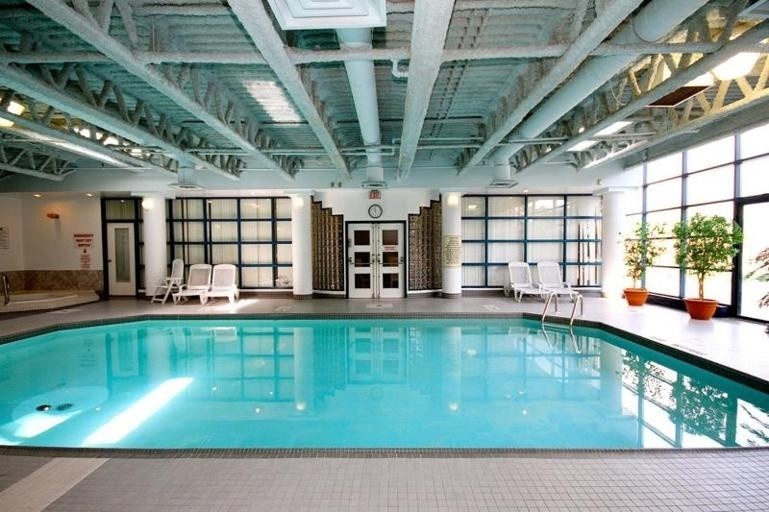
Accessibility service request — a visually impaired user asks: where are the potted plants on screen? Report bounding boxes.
[622,224,666,307]
[672,211,743,320]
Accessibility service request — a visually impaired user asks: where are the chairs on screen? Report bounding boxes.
[537,260,580,303]
[172,262,212,307]
[203,263,240,305]
[507,261,549,303]
[149,259,186,307]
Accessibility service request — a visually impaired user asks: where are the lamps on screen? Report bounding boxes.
[710,22,768,81]
[0,96,27,128]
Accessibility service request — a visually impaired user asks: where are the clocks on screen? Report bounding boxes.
[368,204,382,218]
